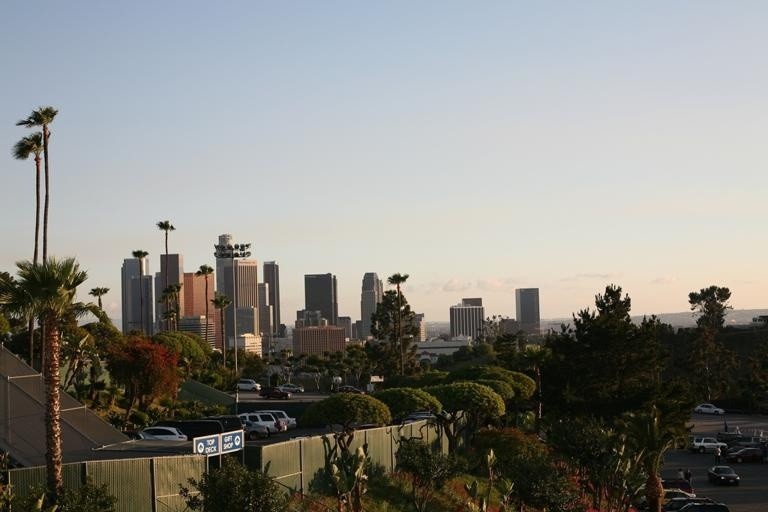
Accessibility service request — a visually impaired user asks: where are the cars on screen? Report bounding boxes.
[259,383,304,400]
[335,385,361,394]
[708,465,740,486]
[688,426,768,463]
[134,409,297,442]
[661,478,730,512]
[694,403,726,415]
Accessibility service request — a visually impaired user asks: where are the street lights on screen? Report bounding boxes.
[213,244,251,377]
[333,375,342,394]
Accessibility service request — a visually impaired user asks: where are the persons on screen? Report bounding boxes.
[678,469,683,480]
[685,468,692,484]
[713,445,721,465]
[721,446,728,464]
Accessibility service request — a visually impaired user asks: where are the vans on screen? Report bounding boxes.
[236,378,261,392]
